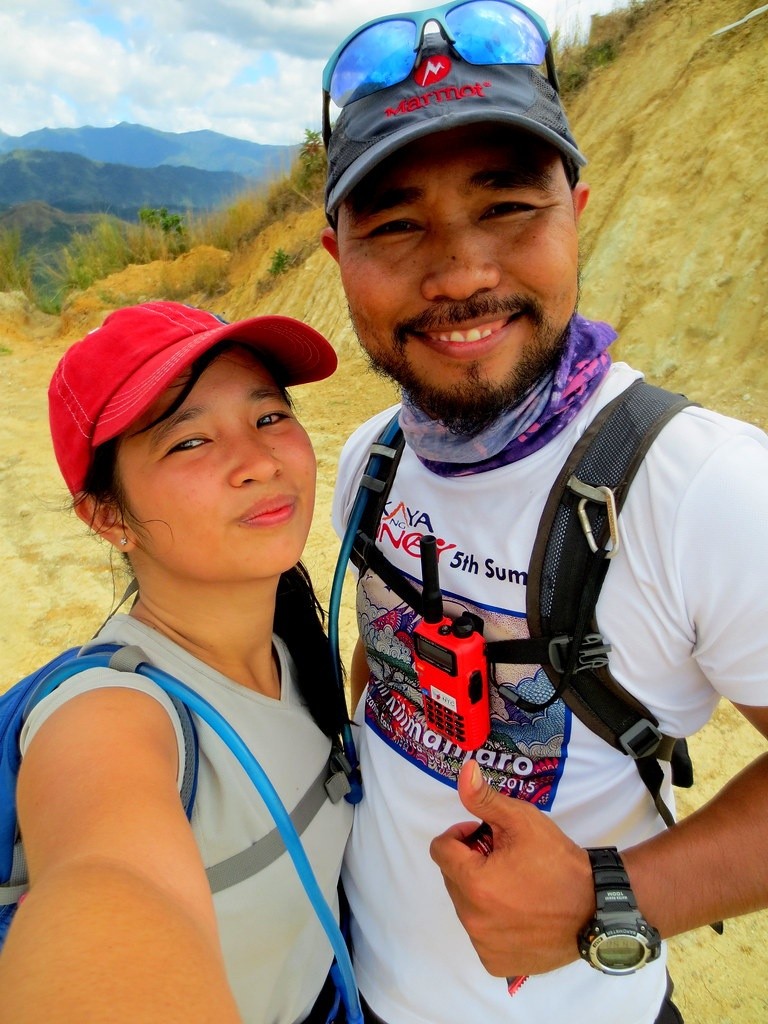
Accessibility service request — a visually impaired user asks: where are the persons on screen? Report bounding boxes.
[320,0,768,1024]
[0,299,363,1024]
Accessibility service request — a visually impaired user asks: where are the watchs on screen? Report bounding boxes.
[575,846,660,976]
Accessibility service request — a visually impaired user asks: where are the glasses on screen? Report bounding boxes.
[322,0,559,158]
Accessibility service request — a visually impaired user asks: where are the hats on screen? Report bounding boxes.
[325,32,588,233]
[49,301,338,494]
